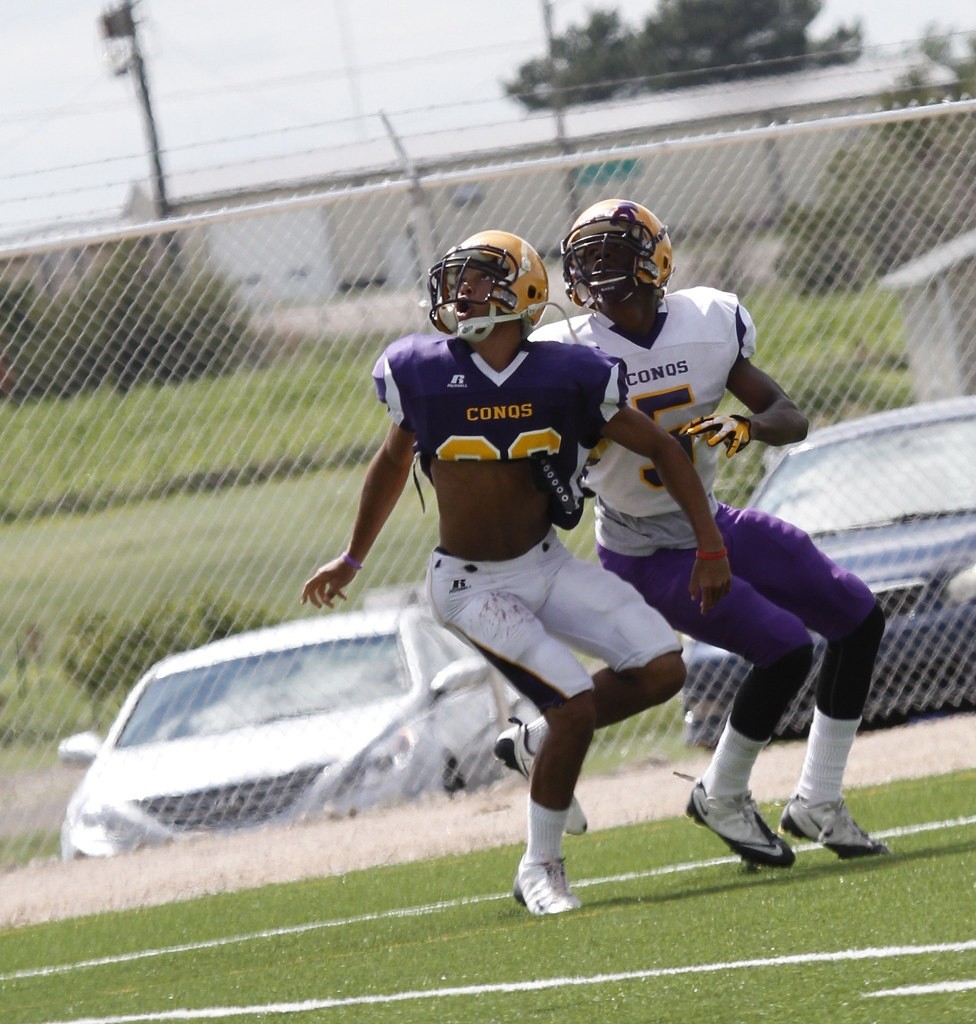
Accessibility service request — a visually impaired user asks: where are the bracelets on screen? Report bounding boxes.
[340,552,362,569]
[696,548,728,560]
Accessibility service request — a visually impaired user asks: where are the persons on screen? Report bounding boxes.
[520,199,889,870]
[301,229,732,916]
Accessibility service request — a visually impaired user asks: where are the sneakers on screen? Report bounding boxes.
[685,777,796,868]
[492,717,587,836]
[778,792,888,859]
[511,852,582,917]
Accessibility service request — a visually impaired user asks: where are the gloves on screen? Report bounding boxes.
[678,414,752,458]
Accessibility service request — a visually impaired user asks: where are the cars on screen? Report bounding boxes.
[679,394,974,752]
[50,595,541,863]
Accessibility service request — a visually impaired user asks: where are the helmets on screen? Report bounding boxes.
[431,228,549,334]
[564,198,672,308]
